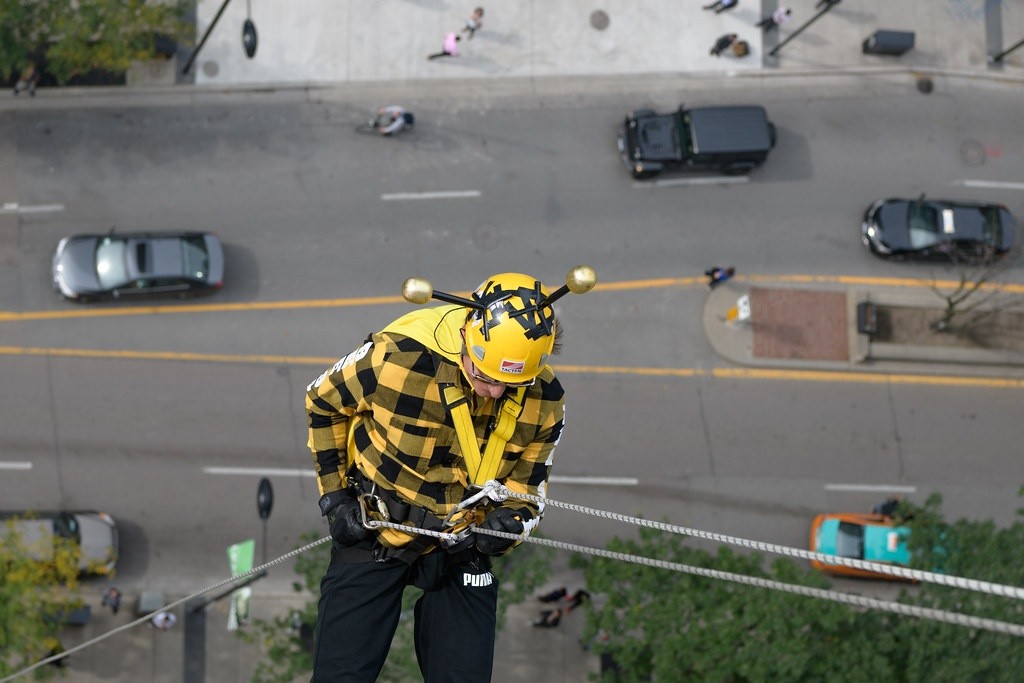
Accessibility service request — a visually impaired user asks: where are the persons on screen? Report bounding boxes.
[871,499,899,515]
[379,105,405,137]
[705,266,736,289]
[582,630,609,653]
[564,590,590,615]
[428,33,461,62]
[147,612,177,632]
[46,648,68,669]
[306,273,566,683]
[539,587,567,603]
[14,59,43,95]
[102,588,121,614]
[703,0,738,15]
[460,7,484,40]
[710,34,736,57]
[529,609,562,628]
[757,6,793,32]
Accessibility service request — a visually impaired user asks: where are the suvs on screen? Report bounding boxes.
[615,101,777,179]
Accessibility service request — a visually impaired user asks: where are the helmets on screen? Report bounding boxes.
[465,272,557,382]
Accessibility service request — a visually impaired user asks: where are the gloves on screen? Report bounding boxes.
[318,486,376,546]
[477,508,524,555]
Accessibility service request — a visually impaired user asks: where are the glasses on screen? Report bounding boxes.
[471,354,536,389]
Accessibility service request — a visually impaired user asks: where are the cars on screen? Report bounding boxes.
[50,224,227,305]
[861,193,1018,269]
[0,510,121,583]
[810,513,952,587]
